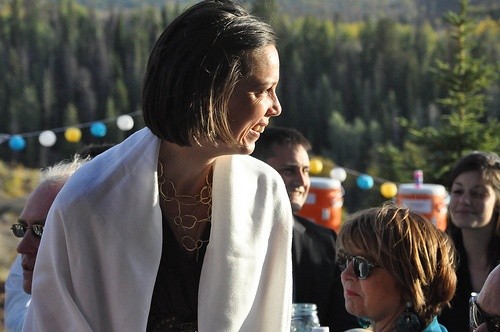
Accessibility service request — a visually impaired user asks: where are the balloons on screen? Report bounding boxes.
[8,114,135,150]
[308,155,398,200]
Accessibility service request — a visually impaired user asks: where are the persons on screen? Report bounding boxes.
[334,207,460,332]
[20,0,294,332]
[466,263,500,332]
[436,149,500,332]
[9,149,95,297]
[250,124,369,332]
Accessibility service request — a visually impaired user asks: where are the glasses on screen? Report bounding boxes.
[469,292,500,332]
[9,223,46,238]
[337,249,381,280]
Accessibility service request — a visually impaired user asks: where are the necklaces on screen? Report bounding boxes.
[153,162,214,253]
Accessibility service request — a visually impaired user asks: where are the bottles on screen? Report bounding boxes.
[311,327,329,332]
[290,304,320,332]
